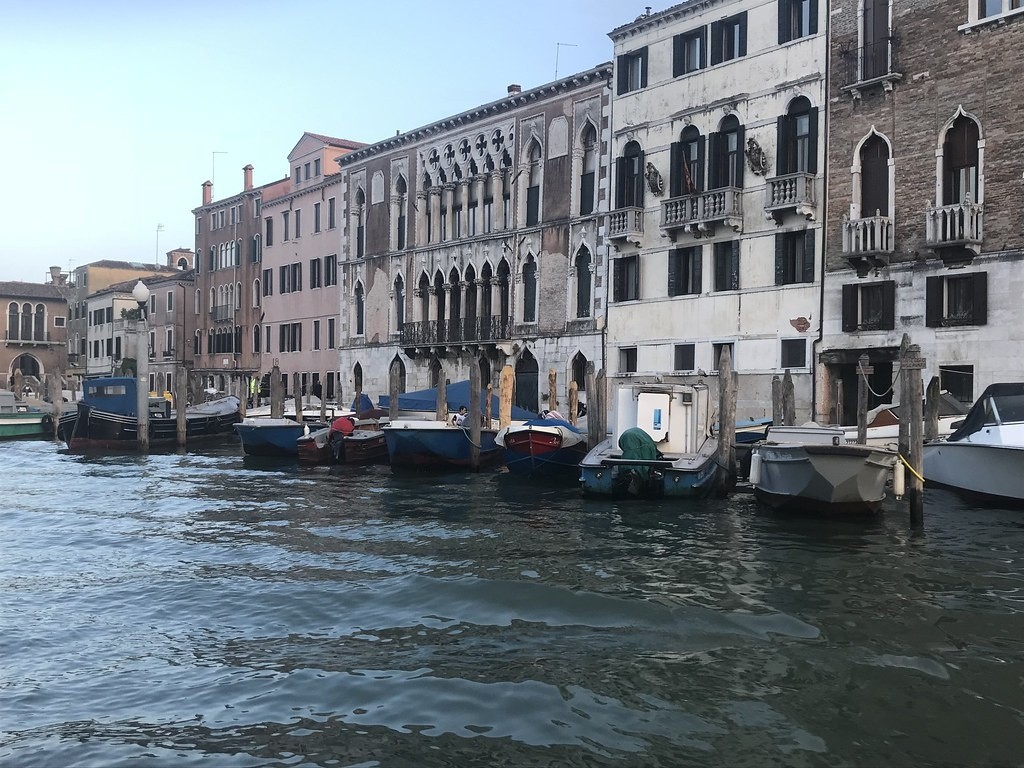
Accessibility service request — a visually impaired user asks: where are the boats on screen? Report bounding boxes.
[381,419,535,470]
[579,382,721,501]
[60,377,239,450]
[0,410,79,440]
[908,382,1024,499]
[803,390,972,453]
[503,416,589,476]
[232,412,356,454]
[295,417,389,466]
[749,427,897,516]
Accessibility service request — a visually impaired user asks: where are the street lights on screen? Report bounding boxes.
[132,281,151,447]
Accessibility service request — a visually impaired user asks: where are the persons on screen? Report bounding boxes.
[315,381,322,401]
[451,406,468,427]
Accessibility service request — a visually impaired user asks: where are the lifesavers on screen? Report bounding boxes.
[57,425,65,441]
[41,414,53,430]
